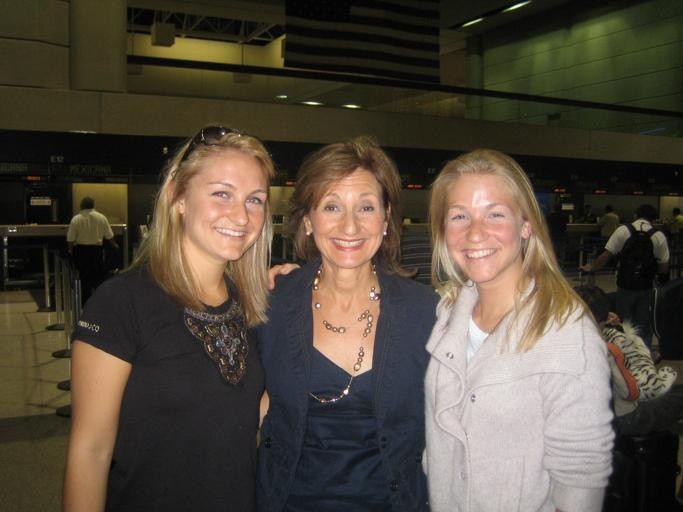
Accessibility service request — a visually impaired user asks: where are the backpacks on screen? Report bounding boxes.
[616,223,660,291]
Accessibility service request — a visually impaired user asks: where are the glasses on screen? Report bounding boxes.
[178,125,269,167]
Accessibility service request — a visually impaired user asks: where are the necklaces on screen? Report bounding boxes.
[306,262,380,409]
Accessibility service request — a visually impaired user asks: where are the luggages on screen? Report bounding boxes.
[648,264,682,360]
[571,270,610,325]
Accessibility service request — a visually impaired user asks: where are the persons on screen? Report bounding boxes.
[418,145,615,512]
[664,205,682,246]
[576,283,683,435]
[580,202,672,363]
[261,134,443,511]
[59,124,301,508]
[66,195,123,309]
[583,204,619,274]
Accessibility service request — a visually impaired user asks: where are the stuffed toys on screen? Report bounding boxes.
[598,317,677,403]
